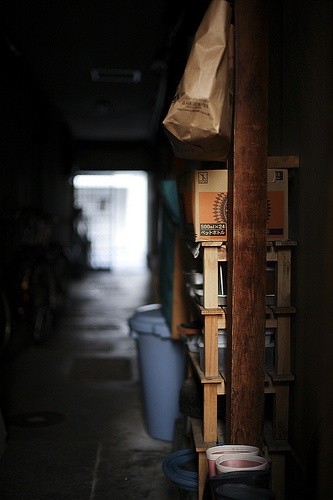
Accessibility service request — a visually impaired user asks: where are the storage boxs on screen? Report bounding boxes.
[184,168,289,246]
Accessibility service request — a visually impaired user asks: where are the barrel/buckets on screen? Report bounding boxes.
[129,309,188,441]
[206,445,267,478]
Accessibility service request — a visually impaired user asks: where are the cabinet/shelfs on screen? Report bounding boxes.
[156,146,297,499]
